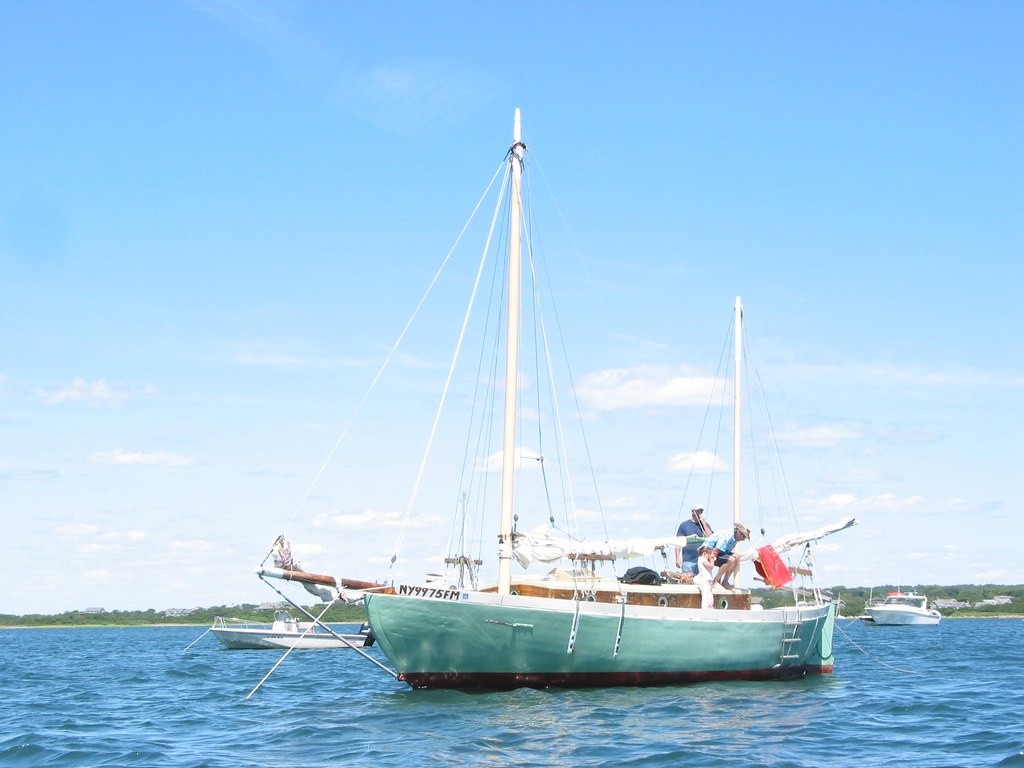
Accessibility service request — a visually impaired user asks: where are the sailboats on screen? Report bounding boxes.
[255,105,856,689]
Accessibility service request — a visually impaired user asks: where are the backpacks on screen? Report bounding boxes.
[617,566,662,586]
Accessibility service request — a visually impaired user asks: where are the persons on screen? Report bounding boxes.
[699,521,751,590]
[675,504,715,582]
[697,547,716,610]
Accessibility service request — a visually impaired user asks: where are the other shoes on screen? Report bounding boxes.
[721,580,735,592]
[712,577,726,591]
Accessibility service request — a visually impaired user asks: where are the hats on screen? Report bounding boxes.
[691,504,705,511]
[736,522,751,541]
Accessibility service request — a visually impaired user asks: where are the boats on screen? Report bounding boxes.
[210,609,376,649]
[862,585,940,625]
